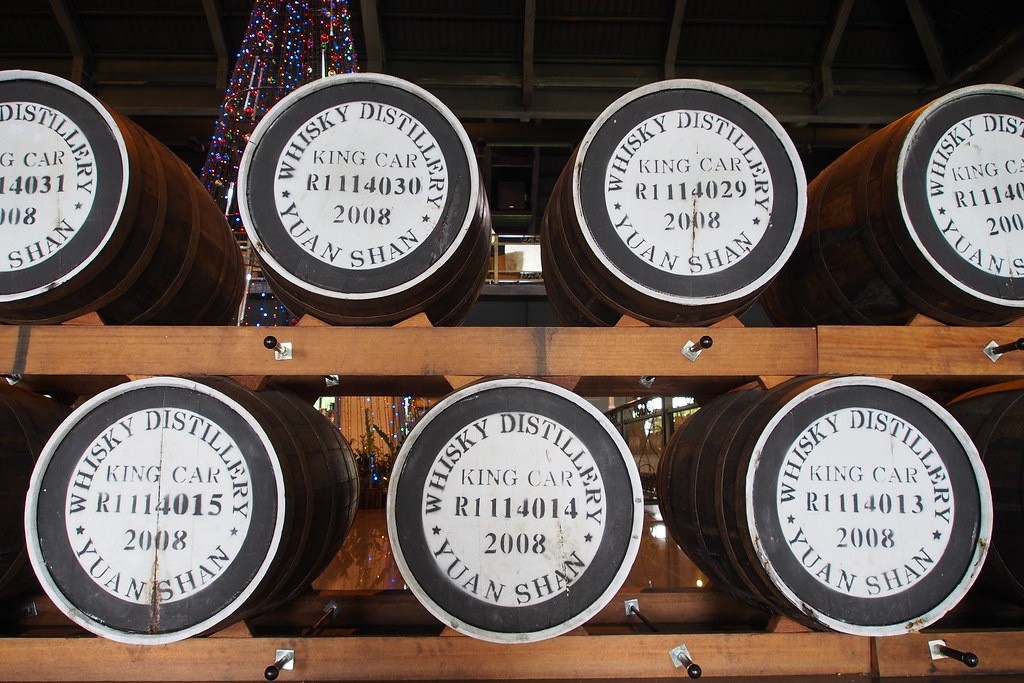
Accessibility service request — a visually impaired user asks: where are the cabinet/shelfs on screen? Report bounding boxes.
[0,325,1024,683]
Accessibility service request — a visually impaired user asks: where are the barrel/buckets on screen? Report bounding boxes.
[936,385,1024,633]
[541,78,808,327]
[27,372,363,646]
[757,83,1024,327]
[383,377,646,645]
[655,374,995,638]
[0,64,244,327]
[0,383,86,600]
[235,72,493,326]
